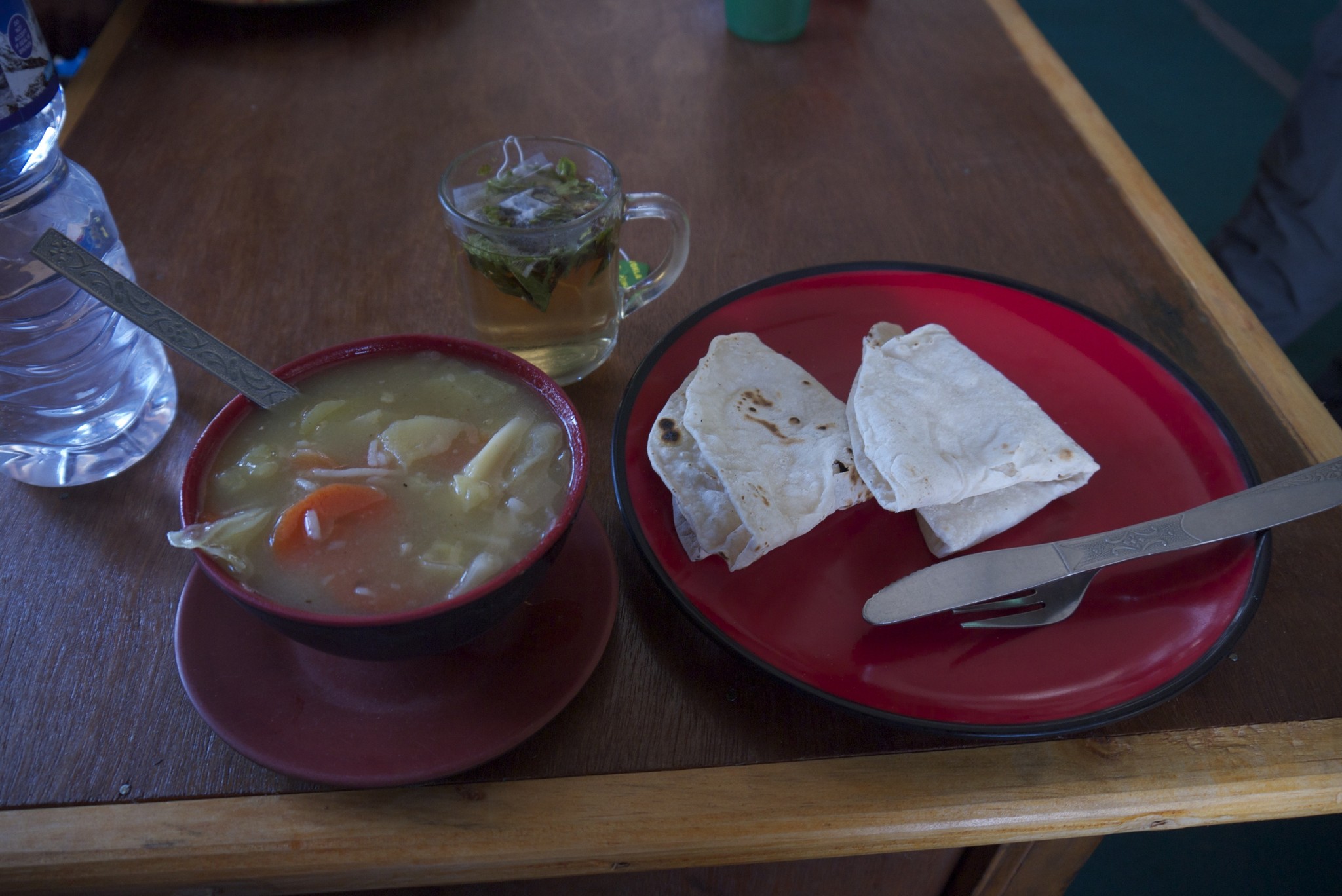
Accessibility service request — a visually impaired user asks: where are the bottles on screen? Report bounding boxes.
[0,0,179,488]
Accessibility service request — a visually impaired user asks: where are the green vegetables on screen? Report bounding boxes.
[459,157,619,309]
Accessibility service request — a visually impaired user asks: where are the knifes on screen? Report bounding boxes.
[862,455,1342,628]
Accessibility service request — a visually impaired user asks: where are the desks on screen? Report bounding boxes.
[0,0,1342,896]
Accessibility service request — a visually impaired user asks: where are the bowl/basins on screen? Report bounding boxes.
[179,333,592,663]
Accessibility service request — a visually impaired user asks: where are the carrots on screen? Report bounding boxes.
[269,482,410,610]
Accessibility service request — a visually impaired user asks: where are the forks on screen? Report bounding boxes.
[952,567,1101,628]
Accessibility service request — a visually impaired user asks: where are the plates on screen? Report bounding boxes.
[612,260,1273,740]
[174,496,621,788]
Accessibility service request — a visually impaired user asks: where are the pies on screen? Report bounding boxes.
[646,332,877,572]
[842,321,1104,555]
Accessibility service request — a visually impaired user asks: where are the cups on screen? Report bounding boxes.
[724,0,810,42]
[438,137,690,387]
